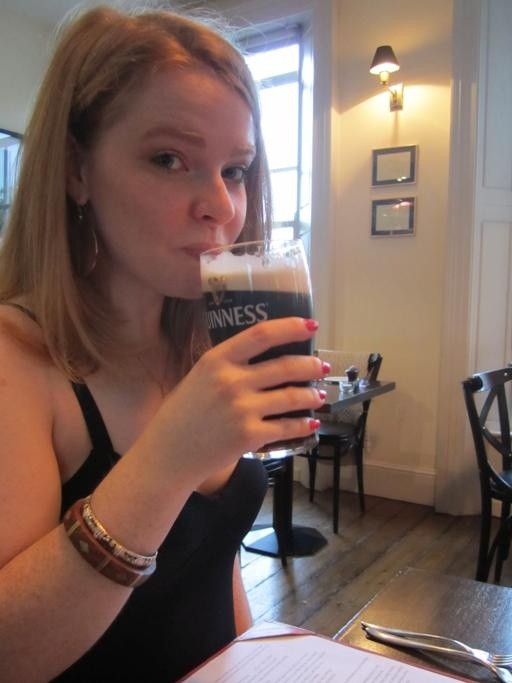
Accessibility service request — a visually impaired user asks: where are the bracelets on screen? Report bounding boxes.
[82,491,160,569]
[63,497,158,588]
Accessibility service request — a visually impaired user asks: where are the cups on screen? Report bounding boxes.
[198,237,320,461]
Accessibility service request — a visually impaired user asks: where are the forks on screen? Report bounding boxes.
[360,619,512,667]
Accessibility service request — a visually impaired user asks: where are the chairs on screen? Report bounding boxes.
[462,363,510,585]
[307,353,382,534]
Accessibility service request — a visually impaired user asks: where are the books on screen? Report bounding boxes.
[175,618,478,682]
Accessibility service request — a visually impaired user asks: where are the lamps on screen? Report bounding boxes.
[369,46,403,110]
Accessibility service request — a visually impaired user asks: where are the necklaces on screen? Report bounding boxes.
[136,340,174,400]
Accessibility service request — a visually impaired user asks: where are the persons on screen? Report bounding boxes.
[0,2,332,682]
